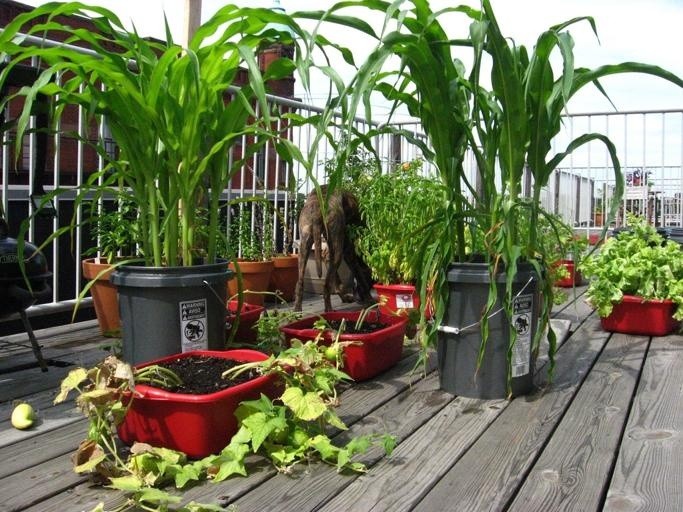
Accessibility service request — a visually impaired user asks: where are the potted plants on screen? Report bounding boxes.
[1,0,682,461]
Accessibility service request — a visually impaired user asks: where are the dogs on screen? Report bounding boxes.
[292,183,378,319]
